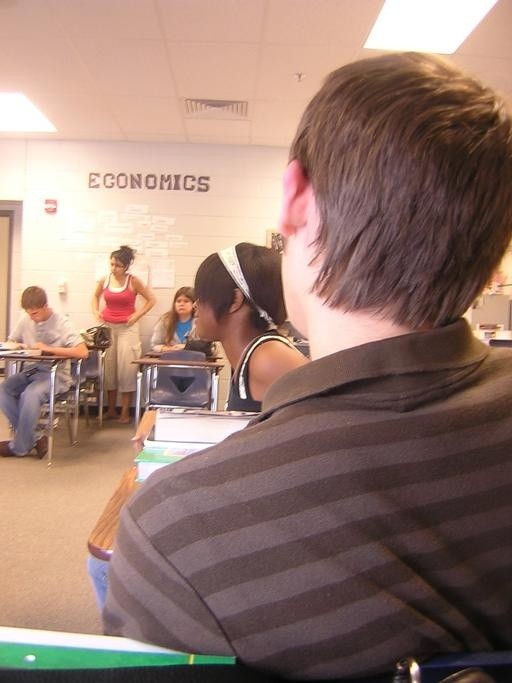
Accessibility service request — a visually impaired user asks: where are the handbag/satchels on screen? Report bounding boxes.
[79,324,115,350]
[183,334,217,359]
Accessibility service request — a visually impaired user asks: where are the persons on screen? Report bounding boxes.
[90,242,157,425]
[149,285,228,363]
[88,240,313,607]
[0,284,91,461]
[96,50,511,681]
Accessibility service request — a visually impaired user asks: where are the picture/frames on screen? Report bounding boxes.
[265,226,287,257]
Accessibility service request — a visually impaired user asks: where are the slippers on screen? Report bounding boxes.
[96,410,119,420]
[117,414,133,423]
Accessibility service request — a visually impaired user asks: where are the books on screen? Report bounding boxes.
[131,406,255,486]
[0,340,42,357]
[0,623,239,672]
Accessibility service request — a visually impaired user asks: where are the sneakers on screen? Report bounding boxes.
[0,439,21,457]
[35,434,50,460]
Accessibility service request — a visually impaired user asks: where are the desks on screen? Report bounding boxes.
[0,341,107,462]
[131,343,220,435]
[87,465,145,615]
